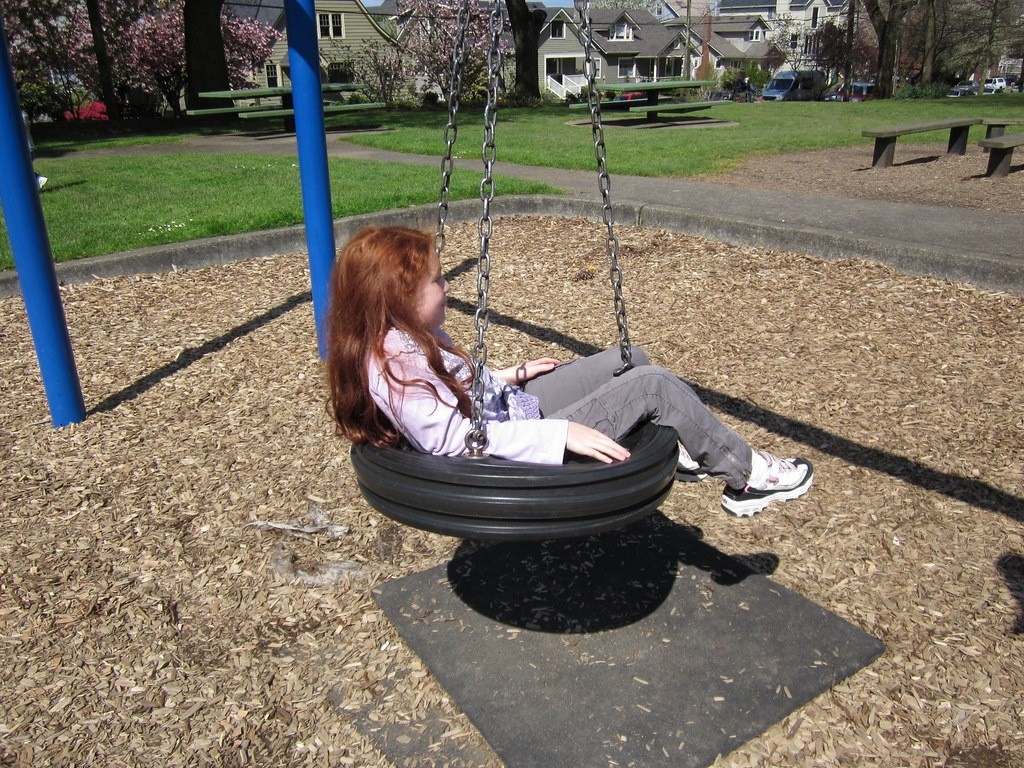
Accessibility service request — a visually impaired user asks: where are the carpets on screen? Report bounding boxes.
[368,514,888,767]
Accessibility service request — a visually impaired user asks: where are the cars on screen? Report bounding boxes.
[953,80,980,91]
[946,89,975,98]
[825,82,876,102]
[975,88,1004,95]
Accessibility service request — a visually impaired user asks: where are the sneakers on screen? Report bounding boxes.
[720,450,814,517]
[674,444,708,483]
[36,176,48,192]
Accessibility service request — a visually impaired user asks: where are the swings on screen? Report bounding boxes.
[349,0,681,547]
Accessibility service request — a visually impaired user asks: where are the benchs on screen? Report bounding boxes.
[861,116,1024,178]
[568,95,725,112]
[185,99,386,120]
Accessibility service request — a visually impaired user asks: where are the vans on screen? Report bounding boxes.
[761,69,826,102]
[984,78,1007,90]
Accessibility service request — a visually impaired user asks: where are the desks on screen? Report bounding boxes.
[586,80,719,123]
[198,82,370,135]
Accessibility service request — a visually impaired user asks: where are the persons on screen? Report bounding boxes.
[327,226,813,517]
